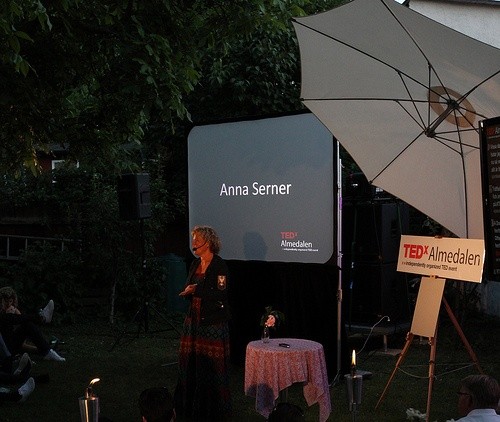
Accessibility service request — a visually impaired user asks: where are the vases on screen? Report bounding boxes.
[263,326,270,342]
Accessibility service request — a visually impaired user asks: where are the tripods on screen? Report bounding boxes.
[110,220,176,351]
[374,276,486,422]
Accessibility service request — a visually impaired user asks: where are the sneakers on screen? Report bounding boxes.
[17,377,35,403]
[43,349,65,361]
[39,299,54,323]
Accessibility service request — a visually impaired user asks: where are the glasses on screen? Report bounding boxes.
[456,392,473,403]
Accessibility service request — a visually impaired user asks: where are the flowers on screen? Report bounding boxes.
[260,306,281,337]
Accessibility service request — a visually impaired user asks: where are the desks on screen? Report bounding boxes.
[244,338,331,422]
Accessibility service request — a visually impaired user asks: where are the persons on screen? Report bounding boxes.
[268,402,305,422]
[138,387,176,422]
[453,375,500,422]
[176,226,233,422]
[0,286,65,405]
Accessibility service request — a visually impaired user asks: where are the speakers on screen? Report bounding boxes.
[341,201,411,264]
[342,264,409,328]
[117,173,152,219]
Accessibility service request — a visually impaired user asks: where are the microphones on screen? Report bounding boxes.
[192,242,206,251]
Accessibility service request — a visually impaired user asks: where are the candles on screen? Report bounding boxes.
[352,349,356,378]
[85,378,100,399]
